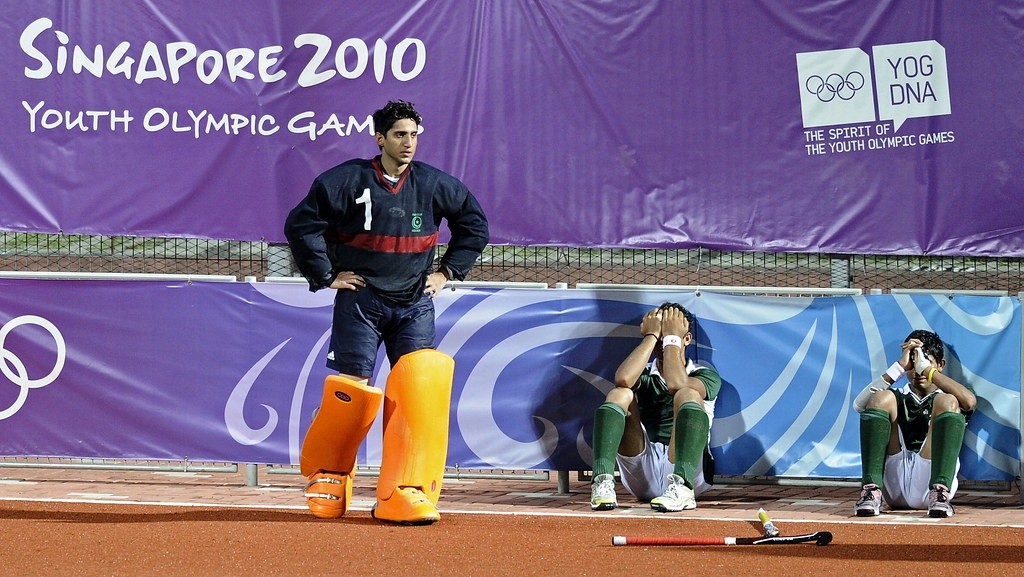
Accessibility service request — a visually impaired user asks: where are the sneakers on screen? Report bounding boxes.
[652,483,698,511]
[855,483,883,518]
[589,478,617,510]
[928,484,955,518]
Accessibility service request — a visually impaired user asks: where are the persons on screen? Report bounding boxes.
[590,301,721,513]
[851,329,977,516]
[282,97,490,520]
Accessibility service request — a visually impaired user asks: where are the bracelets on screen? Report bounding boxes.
[928,368,937,384]
[644,333,658,342]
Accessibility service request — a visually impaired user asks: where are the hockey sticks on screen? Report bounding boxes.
[611,532,832,546]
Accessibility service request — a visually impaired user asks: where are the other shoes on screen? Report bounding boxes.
[300,348,455,526]
